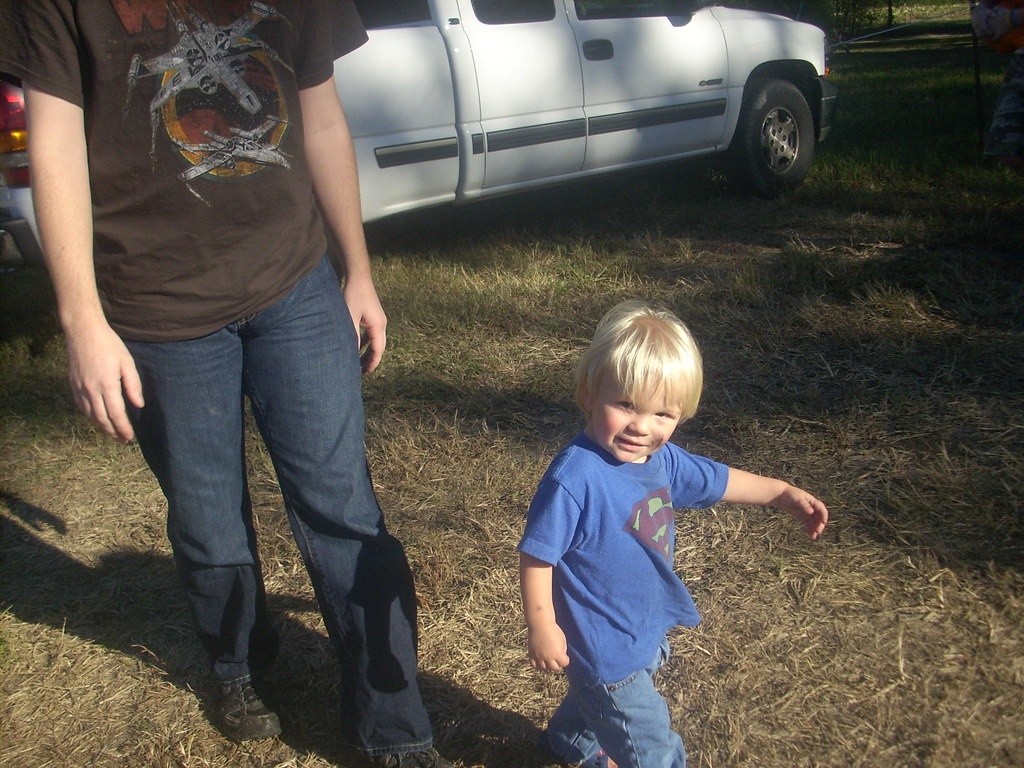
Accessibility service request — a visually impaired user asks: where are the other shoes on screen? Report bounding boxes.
[539,729,618,768]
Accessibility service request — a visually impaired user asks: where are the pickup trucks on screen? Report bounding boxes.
[0,0,838,325]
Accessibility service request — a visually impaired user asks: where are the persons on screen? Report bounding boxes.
[516,300,828,768]
[0,0,451,768]
[969,0,1024,170]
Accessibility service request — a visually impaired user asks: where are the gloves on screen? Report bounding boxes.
[971,4,1014,42]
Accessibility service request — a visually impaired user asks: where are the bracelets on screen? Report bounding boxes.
[1005,8,1020,29]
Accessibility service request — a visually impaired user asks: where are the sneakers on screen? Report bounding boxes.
[352,742,454,768]
[218,667,282,740]
